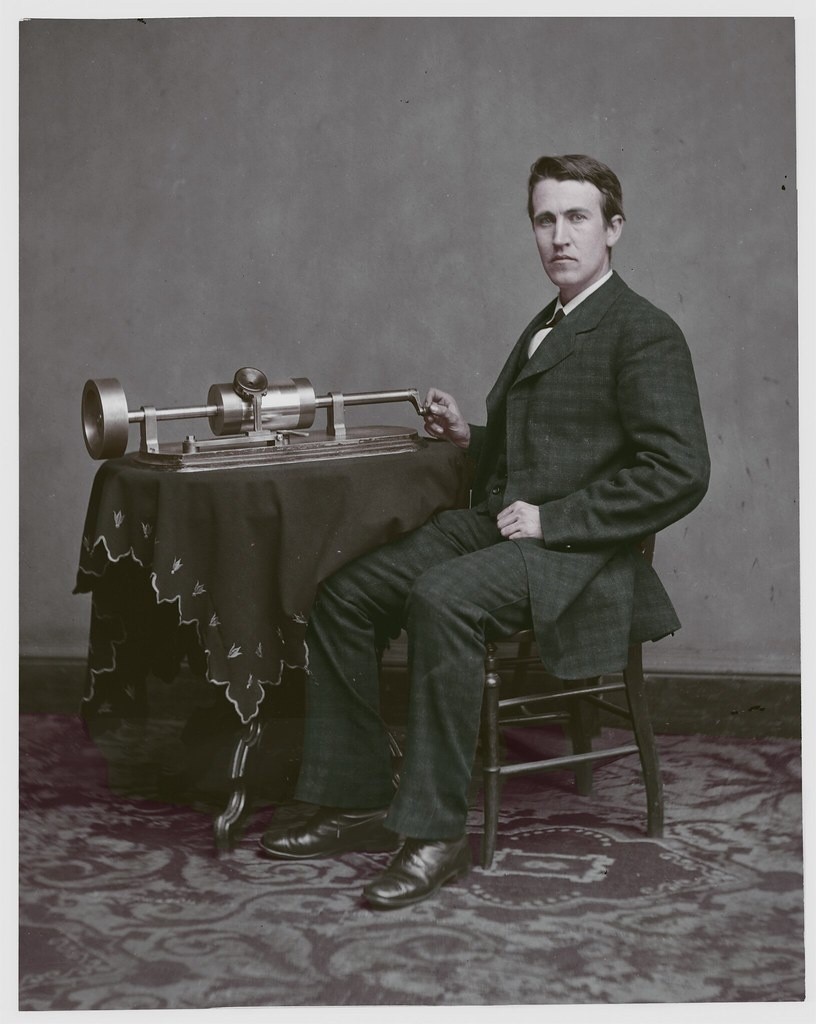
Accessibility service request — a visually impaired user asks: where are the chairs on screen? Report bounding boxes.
[488,531,663,870]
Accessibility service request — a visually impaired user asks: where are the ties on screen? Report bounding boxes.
[544,309,565,328]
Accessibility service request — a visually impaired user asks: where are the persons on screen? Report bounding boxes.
[256,153,712,906]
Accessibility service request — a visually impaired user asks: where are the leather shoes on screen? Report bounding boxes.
[257,807,399,860]
[360,835,472,907]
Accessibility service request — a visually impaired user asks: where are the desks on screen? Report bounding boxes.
[71,436,470,857]
